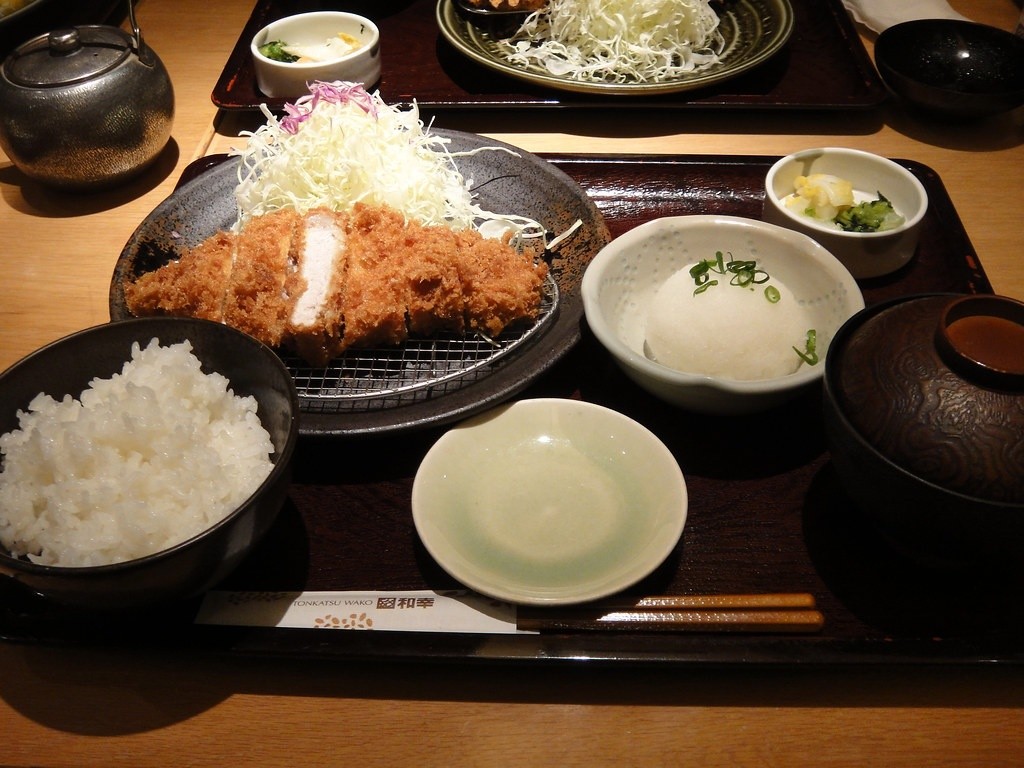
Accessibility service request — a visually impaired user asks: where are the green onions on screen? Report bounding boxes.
[690,249,818,376]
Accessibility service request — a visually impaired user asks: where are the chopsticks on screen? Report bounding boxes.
[519,594,824,631]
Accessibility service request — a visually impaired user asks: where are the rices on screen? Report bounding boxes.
[0,337,275,567]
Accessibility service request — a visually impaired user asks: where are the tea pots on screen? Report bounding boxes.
[0,0,175,192]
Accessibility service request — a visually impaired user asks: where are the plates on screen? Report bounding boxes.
[437,0,795,95]
[411,397,689,606]
[109,126,611,435]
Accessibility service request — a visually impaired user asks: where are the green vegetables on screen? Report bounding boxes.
[803,189,905,233]
[258,38,302,63]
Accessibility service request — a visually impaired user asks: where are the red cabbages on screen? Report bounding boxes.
[280,78,377,136]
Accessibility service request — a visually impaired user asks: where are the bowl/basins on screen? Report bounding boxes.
[825,294,1024,567]
[251,12,381,104]
[0,315,298,607]
[582,215,864,410]
[874,19,1024,125]
[762,148,928,279]
